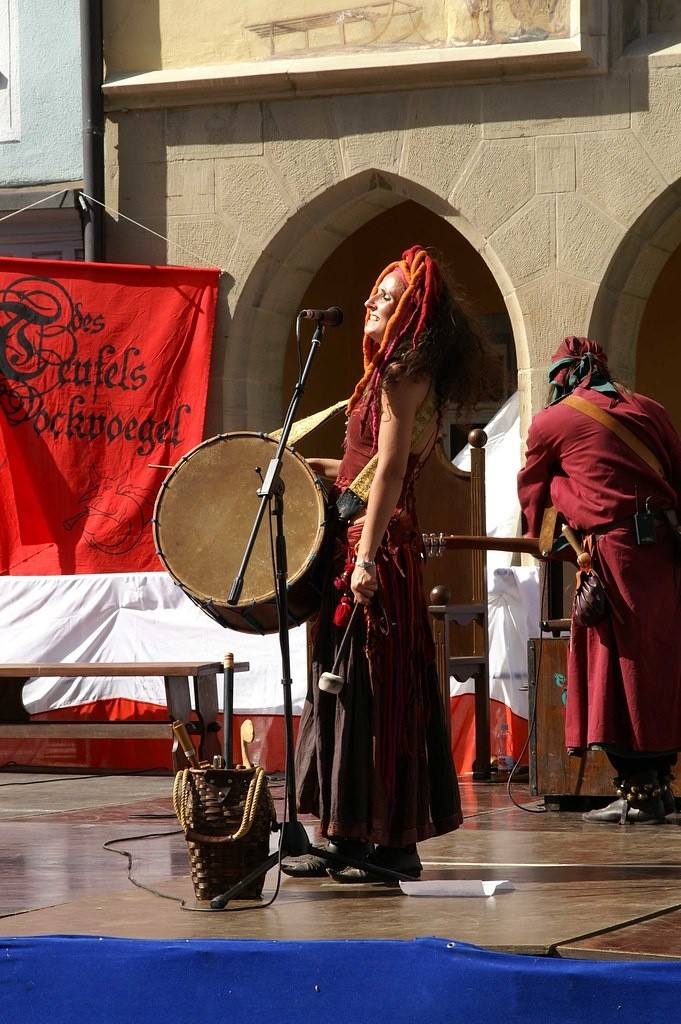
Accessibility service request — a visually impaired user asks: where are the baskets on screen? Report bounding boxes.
[172,766,279,900]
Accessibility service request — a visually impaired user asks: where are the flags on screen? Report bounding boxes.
[0,257,226,576]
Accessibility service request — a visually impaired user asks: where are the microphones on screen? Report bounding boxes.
[297,307,343,327]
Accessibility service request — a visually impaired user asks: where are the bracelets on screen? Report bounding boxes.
[355,561,376,567]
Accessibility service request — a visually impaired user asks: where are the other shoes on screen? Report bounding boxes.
[326,839,423,883]
[658,771,676,815]
[280,836,368,877]
[581,770,665,825]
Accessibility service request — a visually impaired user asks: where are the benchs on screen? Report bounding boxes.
[1,662,250,778]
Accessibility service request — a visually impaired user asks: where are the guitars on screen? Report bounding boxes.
[423,499,588,566]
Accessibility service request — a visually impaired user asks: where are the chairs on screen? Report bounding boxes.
[412,429,491,782]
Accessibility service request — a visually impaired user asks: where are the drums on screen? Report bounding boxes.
[151,432,350,636]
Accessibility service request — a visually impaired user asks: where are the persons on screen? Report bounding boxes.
[280,246,500,885]
[516,336,681,826]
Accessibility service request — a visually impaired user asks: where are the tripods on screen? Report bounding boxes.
[208,318,419,909]
